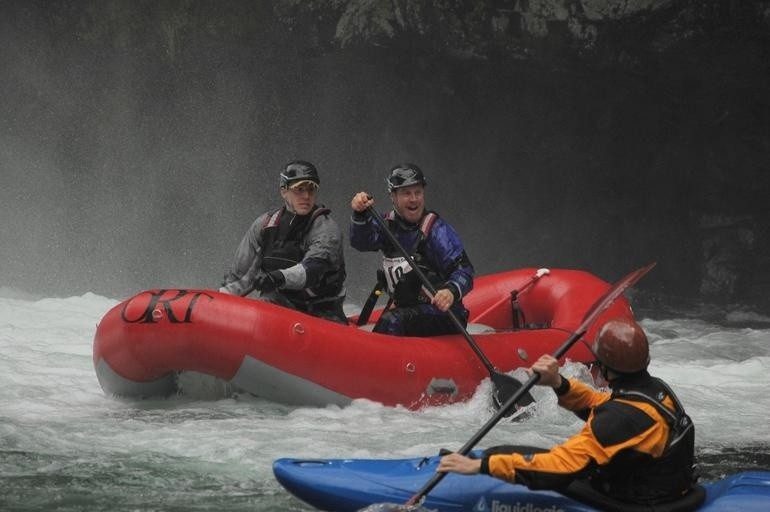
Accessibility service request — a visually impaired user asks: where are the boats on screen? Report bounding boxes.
[92,267,632,408]
[271,449,769,512]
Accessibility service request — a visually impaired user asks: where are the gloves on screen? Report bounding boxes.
[250,271,285,293]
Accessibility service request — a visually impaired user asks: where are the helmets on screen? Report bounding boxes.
[386,163,426,193]
[591,318,650,373]
[280,160,320,188]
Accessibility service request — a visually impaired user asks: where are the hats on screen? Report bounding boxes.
[288,179,320,190]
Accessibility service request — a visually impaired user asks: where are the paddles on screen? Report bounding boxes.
[401,261,656,507]
[366,194,536,421]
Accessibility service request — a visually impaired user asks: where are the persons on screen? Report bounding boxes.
[218,159,350,327]
[435,317,695,501]
[349,164,477,337]
